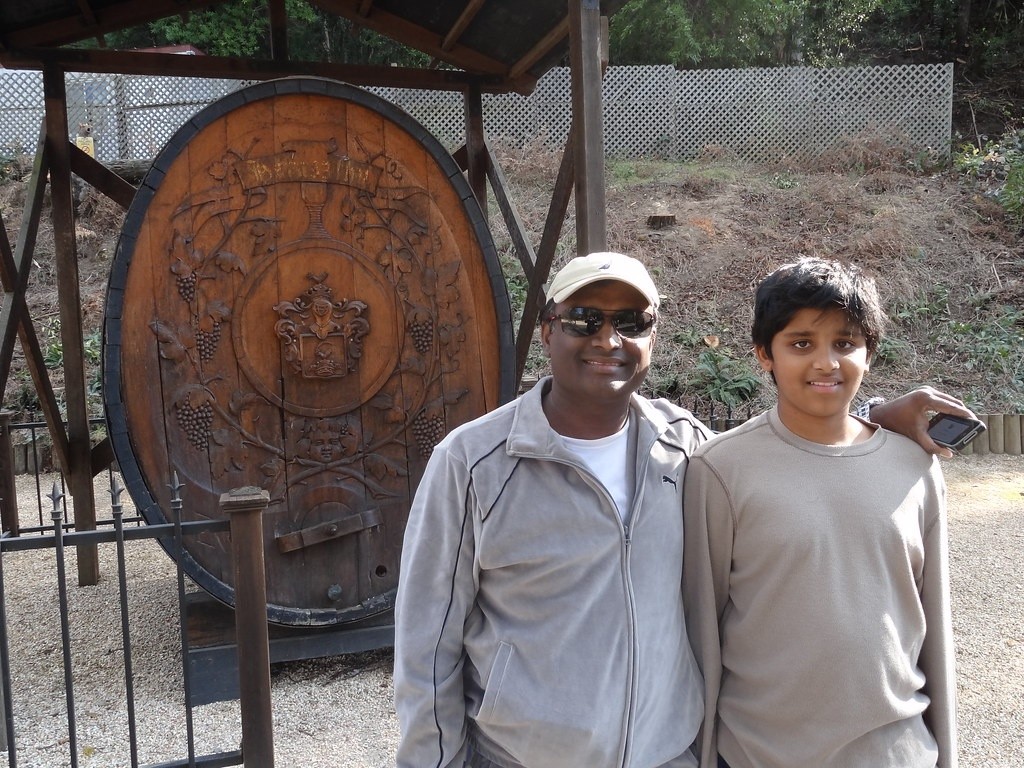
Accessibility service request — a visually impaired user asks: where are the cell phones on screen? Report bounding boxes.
[927,412,987,452]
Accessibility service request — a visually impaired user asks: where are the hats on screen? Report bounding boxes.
[545,251,660,314]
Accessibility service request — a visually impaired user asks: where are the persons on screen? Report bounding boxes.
[392,253,980,768]
[683,260,958,768]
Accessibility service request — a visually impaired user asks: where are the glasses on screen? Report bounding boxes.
[548,306,656,338]
